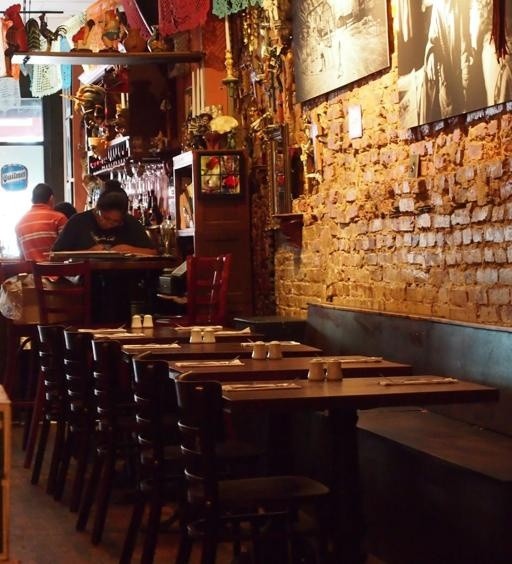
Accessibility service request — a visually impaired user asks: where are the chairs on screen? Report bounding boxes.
[0,247,511,564]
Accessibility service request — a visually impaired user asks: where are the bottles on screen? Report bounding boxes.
[307,358,326,381]
[25,18,41,50]
[265,341,282,359]
[202,328,216,344]
[165,216,175,250]
[188,327,203,343]
[130,314,143,328]
[141,314,154,328]
[324,358,344,380]
[250,340,267,360]
[125,189,161,225]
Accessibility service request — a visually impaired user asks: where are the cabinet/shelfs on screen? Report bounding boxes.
[170,146,255,322]
[74,59,178,177]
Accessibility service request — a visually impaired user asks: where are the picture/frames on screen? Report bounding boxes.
[196,150,244,201]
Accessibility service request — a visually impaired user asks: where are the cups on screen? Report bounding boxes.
[102,144,128,170]
[117,160,167,188]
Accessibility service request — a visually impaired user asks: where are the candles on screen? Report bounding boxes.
[224,12,232,52]
[190,66,205,118]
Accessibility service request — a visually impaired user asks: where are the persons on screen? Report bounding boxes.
[49,179,160,323]
[52,201,77,219]
[16,183,69,263]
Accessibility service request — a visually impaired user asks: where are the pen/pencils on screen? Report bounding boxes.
[90,231,100,246]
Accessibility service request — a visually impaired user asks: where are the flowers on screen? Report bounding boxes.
[185,103,238,136]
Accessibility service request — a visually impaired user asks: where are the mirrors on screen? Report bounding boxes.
[269,123,293,215]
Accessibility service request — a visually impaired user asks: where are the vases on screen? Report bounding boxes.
[122,25,145,53]
[203,131,227,151]
